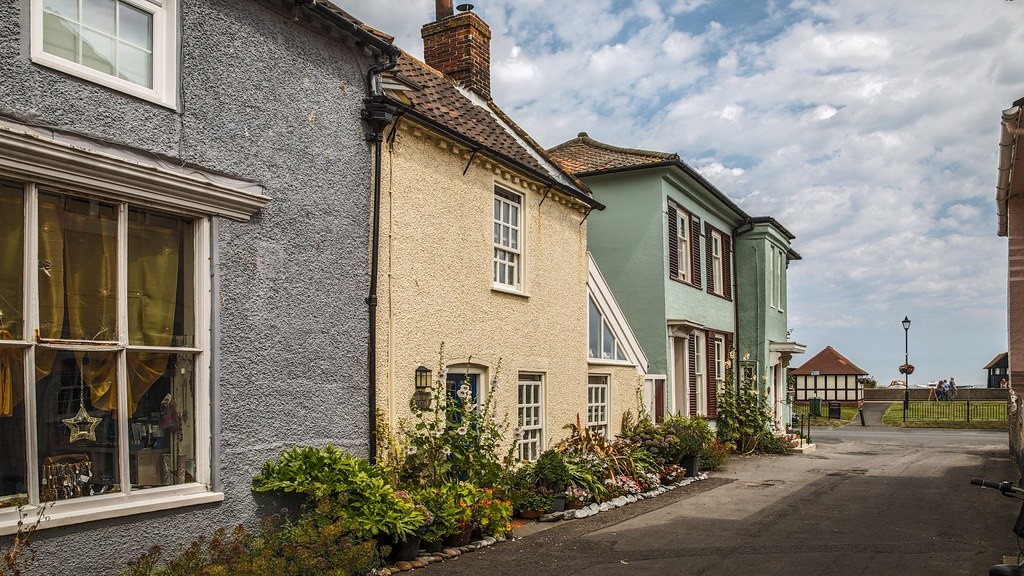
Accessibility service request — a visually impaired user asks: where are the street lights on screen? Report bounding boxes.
[902,315,911,408]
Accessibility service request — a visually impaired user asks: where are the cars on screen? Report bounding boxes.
[792,412,798,422]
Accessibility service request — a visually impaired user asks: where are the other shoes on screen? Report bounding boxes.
[946,399,948,401]
[950,399,953,401]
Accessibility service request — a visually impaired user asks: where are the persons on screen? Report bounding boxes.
[934,379,949,402]
[1000,378,1008,389]
[950,378,957,401]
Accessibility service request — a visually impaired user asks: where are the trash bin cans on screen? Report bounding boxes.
[808,397,823,417]
[828,400,841,419]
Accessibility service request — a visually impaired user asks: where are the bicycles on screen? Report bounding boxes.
[971,477,1024,575]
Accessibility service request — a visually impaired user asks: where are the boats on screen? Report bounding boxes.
[915,381,973,388]
[888,379,909,390]
